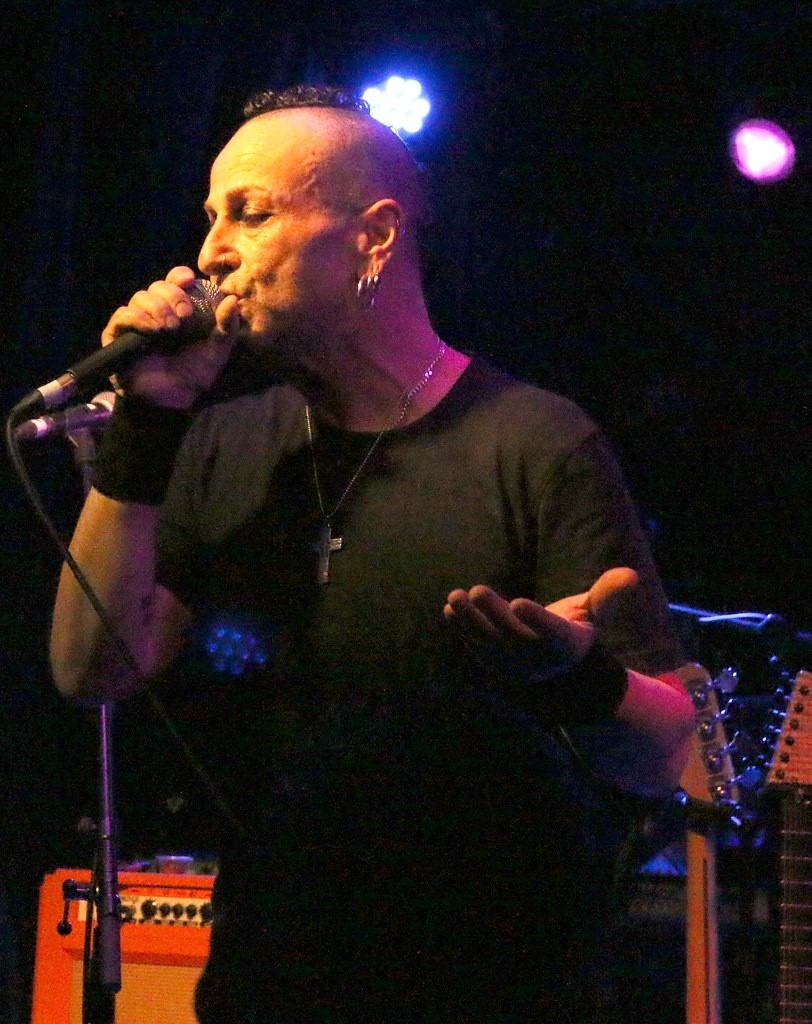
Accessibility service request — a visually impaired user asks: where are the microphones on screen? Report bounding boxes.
[13,390,117,444]
[15,277,225,416]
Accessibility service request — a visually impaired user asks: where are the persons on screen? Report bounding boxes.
[45,87,699,1024]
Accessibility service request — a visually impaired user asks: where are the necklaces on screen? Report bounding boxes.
[302,336,445,584]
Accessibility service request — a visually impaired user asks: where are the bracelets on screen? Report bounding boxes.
[547,640,630,728]
[91,394,197,508]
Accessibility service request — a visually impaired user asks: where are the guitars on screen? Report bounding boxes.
[751,661,812,1024]
[655,659,764,1024]
